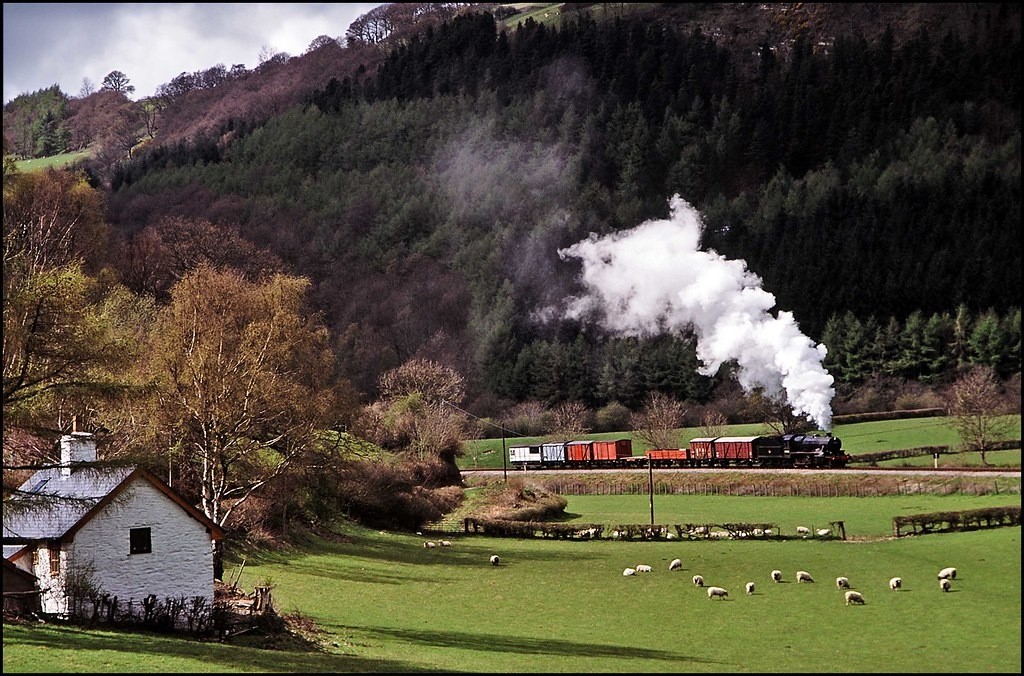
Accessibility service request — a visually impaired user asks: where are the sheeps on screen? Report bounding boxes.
[937,567,957,592]
[844,591,865,607]
[816,528,830,536]
[707,586,728,601]
[622,564,652,576]
[889,577,902,591]
[771,570,782,583]
[796,526,811,535]
[796,570,815,584]
[576,526,774,540]
[489,555,500,567]
[669,558,682,571]
[746,581,755,595]
[423,539,451,549]
[836,577,850,590]
[692,574,705,587]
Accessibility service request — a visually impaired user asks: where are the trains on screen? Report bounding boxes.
[509,432,852,470]
